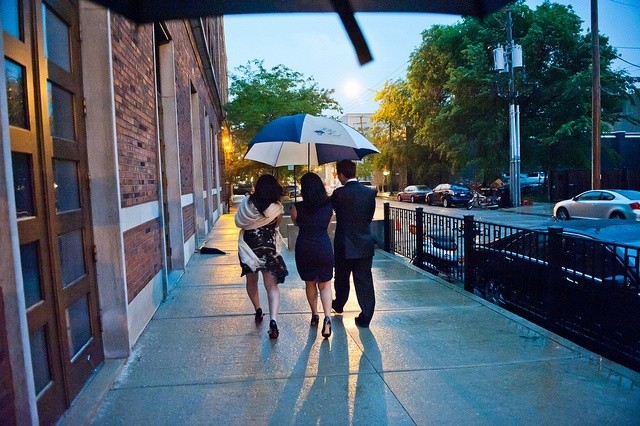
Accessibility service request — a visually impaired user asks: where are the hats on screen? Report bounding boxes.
[359,180,378,195]
[478,220,639,373]
[397,185,431,203]
[283,185,301,197]
[553,189,640,221]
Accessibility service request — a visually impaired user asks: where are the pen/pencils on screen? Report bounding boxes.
[527,171,544,186]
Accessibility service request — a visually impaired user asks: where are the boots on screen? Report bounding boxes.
[425,183,472,207]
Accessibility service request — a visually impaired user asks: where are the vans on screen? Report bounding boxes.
[254,308,265,324]
[322,316,331,340]
[311,315,319,328]
[267,320,279,339]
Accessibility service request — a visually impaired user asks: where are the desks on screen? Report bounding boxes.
[494,12,523,205]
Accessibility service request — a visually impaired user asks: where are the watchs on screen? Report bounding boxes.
[412,226,487,276]
[466,192,493,209]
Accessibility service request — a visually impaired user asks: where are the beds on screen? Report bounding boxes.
[243,113,382,173]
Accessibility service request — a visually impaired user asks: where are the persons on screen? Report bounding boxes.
[330,159,376,328]
[290,173,335,340]
[235,174,289,340]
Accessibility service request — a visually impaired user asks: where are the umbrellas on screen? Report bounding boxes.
[355,316,370,327]
[331,300,343,313]
